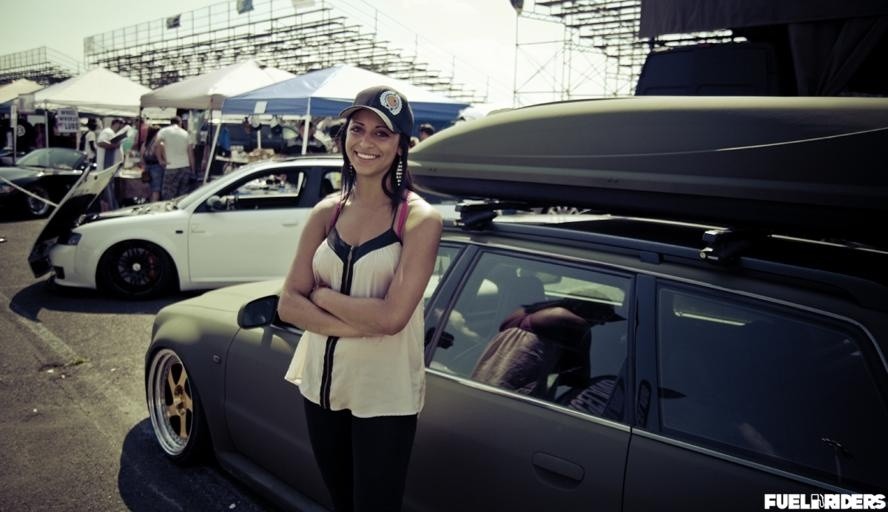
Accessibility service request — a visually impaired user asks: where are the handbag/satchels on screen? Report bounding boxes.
[142,172,151,182]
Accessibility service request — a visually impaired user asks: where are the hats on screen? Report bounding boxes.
[562,287,627,322]
[339,85,415,136]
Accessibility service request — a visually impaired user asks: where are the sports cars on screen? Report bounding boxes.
[0,145,150,219]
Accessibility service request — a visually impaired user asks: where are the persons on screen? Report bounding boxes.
[469,288,628,400]
[278,85,443,512]
[283,119,435,155]
[79,117,195,212]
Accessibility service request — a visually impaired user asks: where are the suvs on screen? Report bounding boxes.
[142,90,888,511]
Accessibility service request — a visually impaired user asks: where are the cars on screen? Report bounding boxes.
[24,148,499,296]
